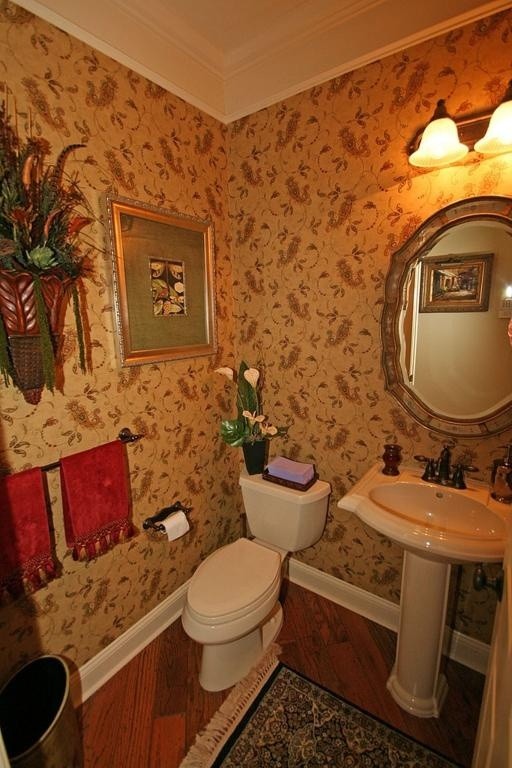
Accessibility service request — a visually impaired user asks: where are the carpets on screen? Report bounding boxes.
[178,644,467,768]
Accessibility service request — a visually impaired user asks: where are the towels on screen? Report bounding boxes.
[0,466,58,608]
[60,441,136,562]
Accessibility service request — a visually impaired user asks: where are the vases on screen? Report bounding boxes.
[0,270,76,404]
[242,439,266,475]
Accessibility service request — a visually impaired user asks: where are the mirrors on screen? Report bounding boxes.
[381,196,512,438]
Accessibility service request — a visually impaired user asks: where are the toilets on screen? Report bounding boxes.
[180,466,332,692]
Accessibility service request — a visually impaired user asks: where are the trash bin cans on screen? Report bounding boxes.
[0,655,78,768]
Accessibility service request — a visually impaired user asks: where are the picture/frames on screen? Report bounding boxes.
[419,254,494,313]
[99,193,219,368]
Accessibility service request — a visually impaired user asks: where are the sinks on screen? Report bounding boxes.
[336,461,512,564]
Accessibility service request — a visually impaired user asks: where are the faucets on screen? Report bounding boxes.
[434,448,451,484]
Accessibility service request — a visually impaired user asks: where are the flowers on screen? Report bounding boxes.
[0,100,121,396]
[213,361,279,447]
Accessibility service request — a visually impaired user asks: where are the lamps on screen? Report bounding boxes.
[406,78,512,168]
